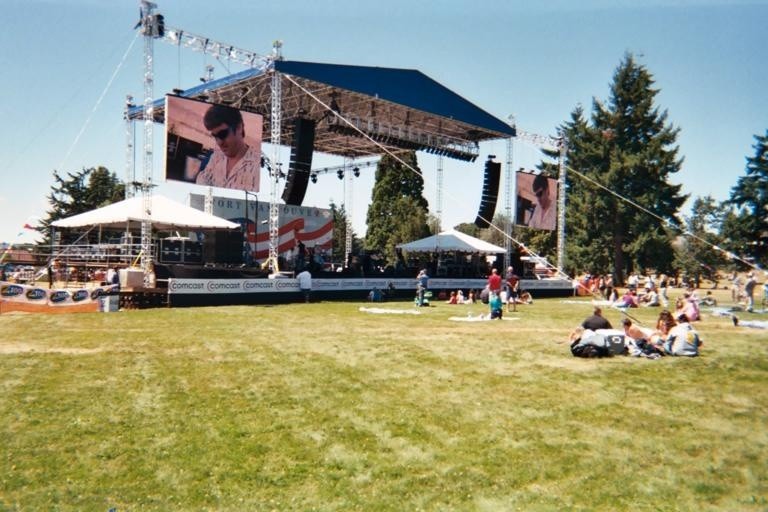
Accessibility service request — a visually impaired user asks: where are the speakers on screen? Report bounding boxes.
[282,117,315,207]
[477,161,502,228]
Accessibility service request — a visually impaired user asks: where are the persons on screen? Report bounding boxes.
[48,265,54,288]
[296,267,311,303]
[368,283,396,303]
[416,266,532,319]
[570,267,768,360]
[296,240,305,270]
[527,176,556,229]
[195,106,260,192]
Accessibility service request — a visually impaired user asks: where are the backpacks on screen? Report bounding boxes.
[570,329,626,357]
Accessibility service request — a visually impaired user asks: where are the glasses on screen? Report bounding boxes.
[212,126,232,140]
